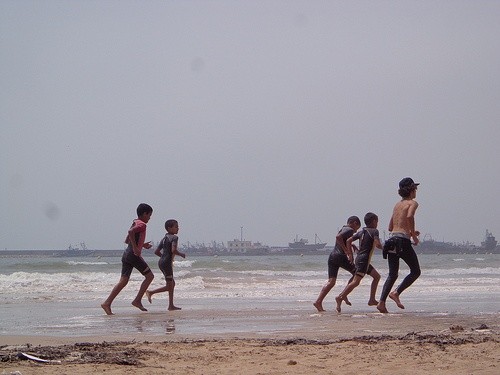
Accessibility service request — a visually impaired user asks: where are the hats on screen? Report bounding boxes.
[399,178,419,189]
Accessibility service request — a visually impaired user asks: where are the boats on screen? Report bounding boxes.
[289,232,327,249]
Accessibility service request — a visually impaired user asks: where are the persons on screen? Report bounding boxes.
[100,203,154,315]
[376,178,422,313]
[312,215,361,312]
[335,212,382,313]
[146,219,185,311]
